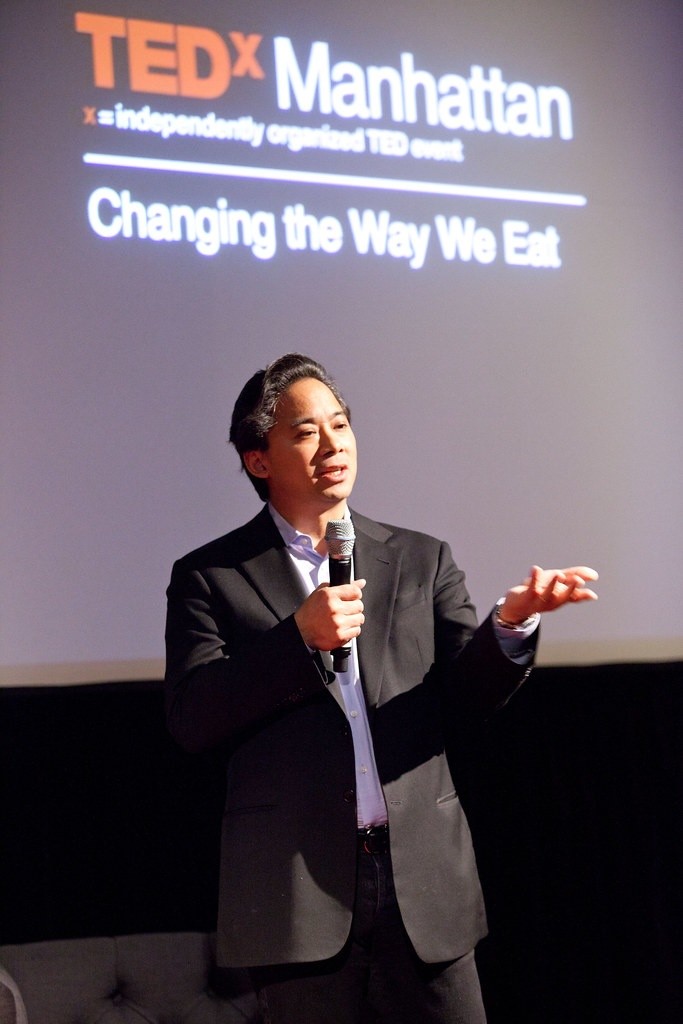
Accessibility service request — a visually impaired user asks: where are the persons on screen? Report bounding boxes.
[159,354,602,1024]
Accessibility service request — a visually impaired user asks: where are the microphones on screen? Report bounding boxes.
[325,519,354,671]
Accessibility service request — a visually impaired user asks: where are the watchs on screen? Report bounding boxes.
[495,596,536,629]
